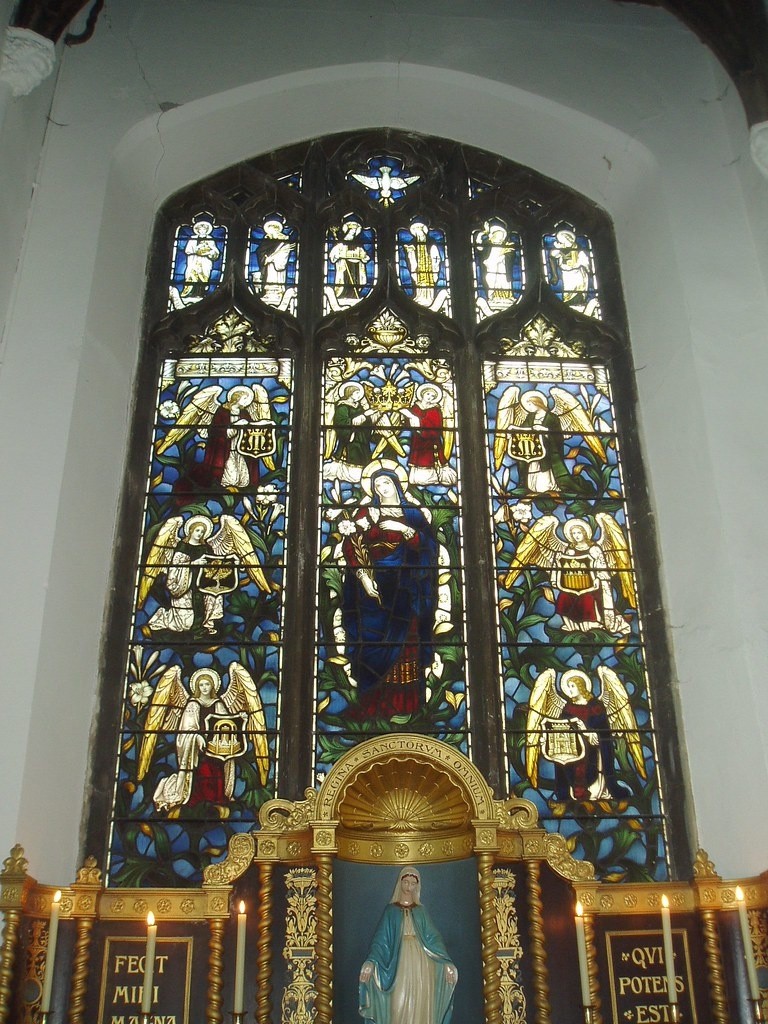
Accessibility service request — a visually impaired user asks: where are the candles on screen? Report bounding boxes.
[41,888,62,1013]
[139,911,157,1013]
[232,901,247,1014]
[661,893,679,1004]
[735,884,760,999]
[574,901,591,1009]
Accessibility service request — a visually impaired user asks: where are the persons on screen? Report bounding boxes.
[357,865,459,1024]
